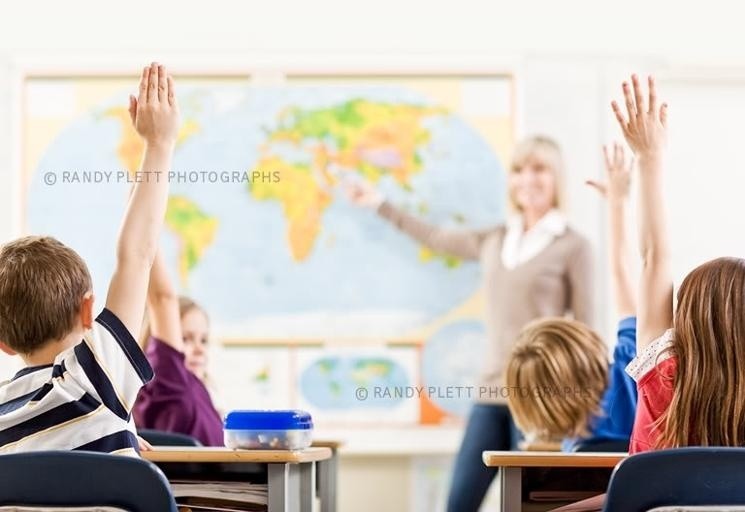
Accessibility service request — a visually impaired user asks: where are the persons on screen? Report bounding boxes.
[508,140,640,451]
[127,243,223,447]
[551,74,743,511]
[342,128,595,512]
[0,61,175,460]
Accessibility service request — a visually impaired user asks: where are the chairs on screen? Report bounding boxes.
[605,448,744,512]
[135,430,204,448]
[0,449,179,512]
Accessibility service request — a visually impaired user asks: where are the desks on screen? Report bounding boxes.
[138,446,334,512]
[482,452,633,512]
[314,440,340,512]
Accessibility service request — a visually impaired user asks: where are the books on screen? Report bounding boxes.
[162,476,268,511]
[525,488,605,503]
